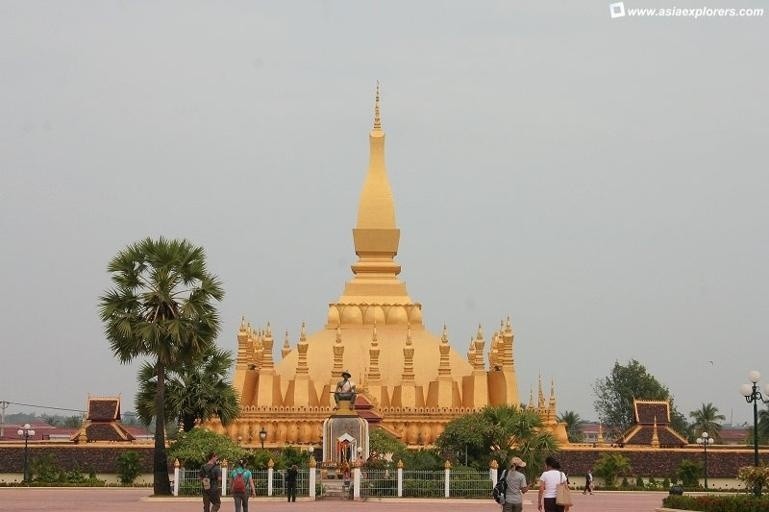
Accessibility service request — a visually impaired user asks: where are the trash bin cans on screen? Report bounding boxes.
[42,435,50,440]
[669,484,684,496]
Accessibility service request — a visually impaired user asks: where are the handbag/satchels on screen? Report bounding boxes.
[556,484,573,506]
[493,479,507,505]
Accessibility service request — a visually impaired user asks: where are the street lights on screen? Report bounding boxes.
[740,369,769,497]
[695,430,716,490]
[16,422,35,482]
[257,427,267,450]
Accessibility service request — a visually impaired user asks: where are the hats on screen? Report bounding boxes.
[510,457,526,467]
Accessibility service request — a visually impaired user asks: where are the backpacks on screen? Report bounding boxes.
[201,466,216,492]
[231,469,246,493]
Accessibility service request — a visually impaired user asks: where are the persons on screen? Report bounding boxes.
[197,451,223,511]
[582,467,594,494]
[537,456,575,511]
[287,465,298,502]
[228,457,257,511]
[329,368,359,410]
[493,456,533,511]
